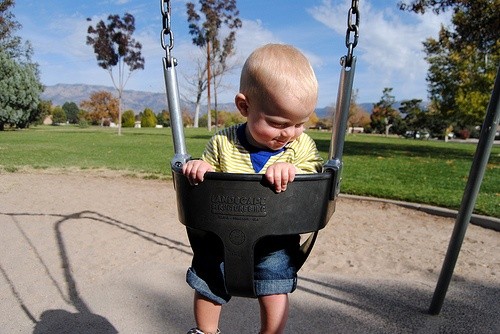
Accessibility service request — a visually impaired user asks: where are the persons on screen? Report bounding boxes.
[182,43,326,334]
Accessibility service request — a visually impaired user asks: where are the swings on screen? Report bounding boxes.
[157,1,362,301]
